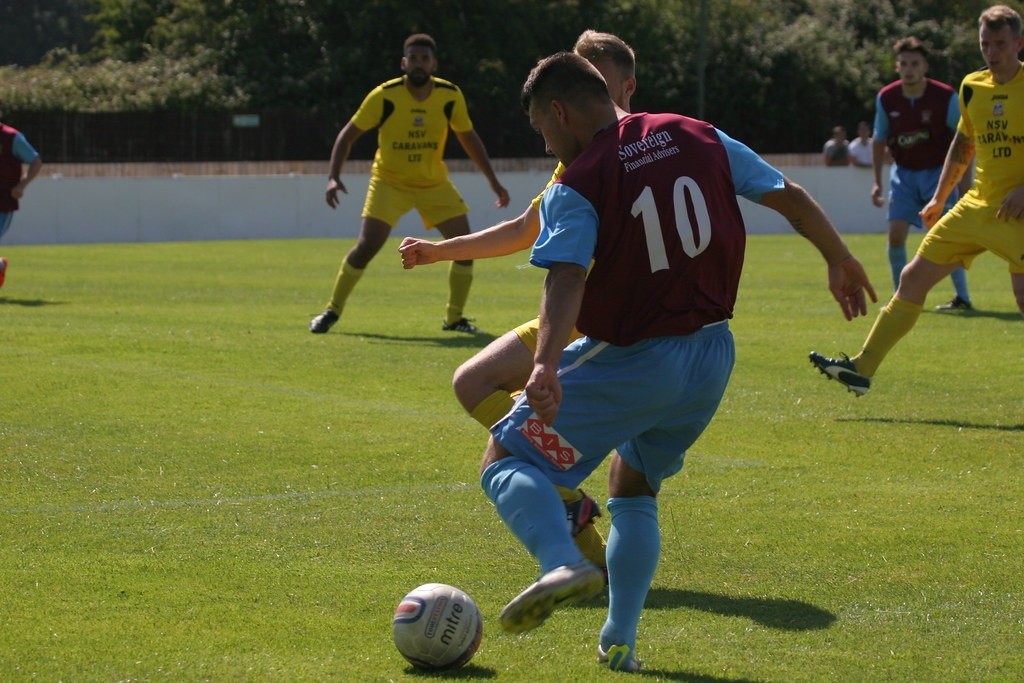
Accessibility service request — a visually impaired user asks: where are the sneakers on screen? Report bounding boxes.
[597,643,639,672]
[882,294,893,309]
[809,350,870,398]
[310,308,339,333]
[499,558,604,634]
[935,295,974,311]
[442,317,477,332]
[597,565,610,586]
[0,257,8,287]
[565,488,602,537]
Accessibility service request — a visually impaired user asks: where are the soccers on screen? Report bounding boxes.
[390,583,483,673]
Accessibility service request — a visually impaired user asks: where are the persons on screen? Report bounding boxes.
[848,121,872,168]
[481,51,876,668]
[809,4,1024,396]
[396,30,636,586]
[0,111,41,286]
[311,34,511,334]
[872,35,972,310]
[824,126,849,166]
